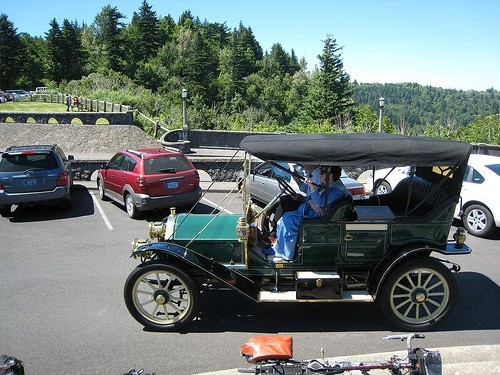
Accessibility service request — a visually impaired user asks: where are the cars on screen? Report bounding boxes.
[0,89,24,103]
[123,131,473,333]
[236,161,365,212]
[365,153,500,238]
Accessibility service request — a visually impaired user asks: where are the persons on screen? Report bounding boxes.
[261,163,348,263]
[64,94,80,111]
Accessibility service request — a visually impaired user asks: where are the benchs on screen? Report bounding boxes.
[300,191,353,246]
[371,176,447,220]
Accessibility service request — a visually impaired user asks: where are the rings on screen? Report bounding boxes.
[308,181,311,183]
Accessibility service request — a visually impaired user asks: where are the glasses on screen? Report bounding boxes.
[321,170,331,175]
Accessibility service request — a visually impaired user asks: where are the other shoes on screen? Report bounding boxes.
[267,253,286,262]
[263,233,277,240]
[263,248,276,254]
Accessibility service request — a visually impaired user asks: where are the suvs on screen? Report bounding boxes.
[0,144,74,217]
[96,146,202,219]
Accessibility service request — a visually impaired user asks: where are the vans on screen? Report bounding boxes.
[35,87,48,93]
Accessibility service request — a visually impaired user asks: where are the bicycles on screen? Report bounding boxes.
[237,332,444,374]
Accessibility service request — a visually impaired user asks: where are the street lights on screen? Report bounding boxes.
[379,95,385,133]
[181,88,189,140]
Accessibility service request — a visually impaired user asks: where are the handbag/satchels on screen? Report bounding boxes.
[412,347,442,375]
